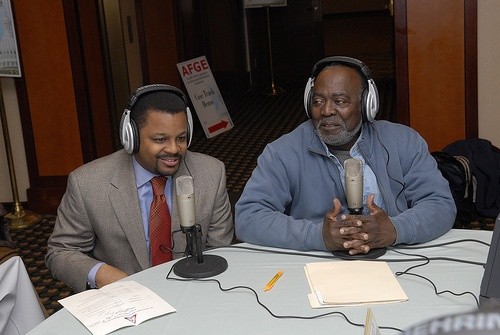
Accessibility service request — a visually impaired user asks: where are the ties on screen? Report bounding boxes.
[149,175,173,267]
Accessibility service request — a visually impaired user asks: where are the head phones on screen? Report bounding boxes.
[304,56,379,123]
[119,84,193,155]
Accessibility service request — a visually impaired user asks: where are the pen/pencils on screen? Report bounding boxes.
[264,271,283,291]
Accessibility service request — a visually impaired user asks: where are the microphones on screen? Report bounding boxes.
[175,174,196,256]
[344,159,364,216]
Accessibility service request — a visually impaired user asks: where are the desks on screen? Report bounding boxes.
[25,228,499,335]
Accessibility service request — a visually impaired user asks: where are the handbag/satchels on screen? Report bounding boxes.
[443,138,500,230]
[426,148,473,230]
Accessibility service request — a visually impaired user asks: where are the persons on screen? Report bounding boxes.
[44,84,234,293]
[234,55,457,255]
